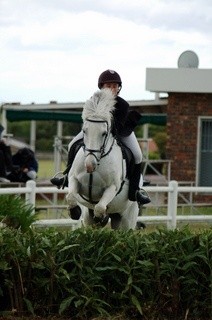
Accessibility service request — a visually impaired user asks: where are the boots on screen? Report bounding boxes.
[49,175,69,186]
[129,164,152,203]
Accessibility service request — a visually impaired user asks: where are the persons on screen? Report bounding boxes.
[50,69,153,205]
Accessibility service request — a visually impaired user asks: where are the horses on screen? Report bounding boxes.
[64,88,144,232]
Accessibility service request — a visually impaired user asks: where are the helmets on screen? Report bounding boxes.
[98,70,121,88]
